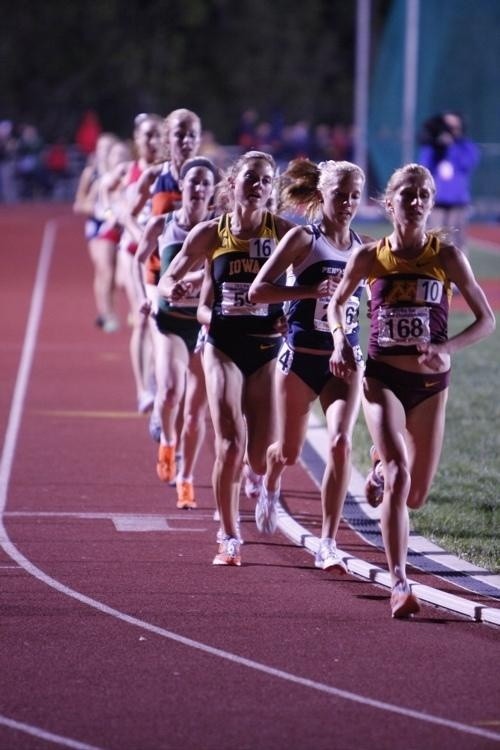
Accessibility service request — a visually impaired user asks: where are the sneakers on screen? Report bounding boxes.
[389,587,421,620]
[364,446,386,507]
[92,312,137,333]
[312,547,348,576]
[137,395,278,566]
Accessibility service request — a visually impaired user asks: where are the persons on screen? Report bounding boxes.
[413,109,485,254]
[323,162,498,622]
[153,149,301,569]
[0,103,379,207]
[73,105,234,510]
[245,156,378,575]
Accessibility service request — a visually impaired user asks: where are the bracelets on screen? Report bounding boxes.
[330,324,345,335]
